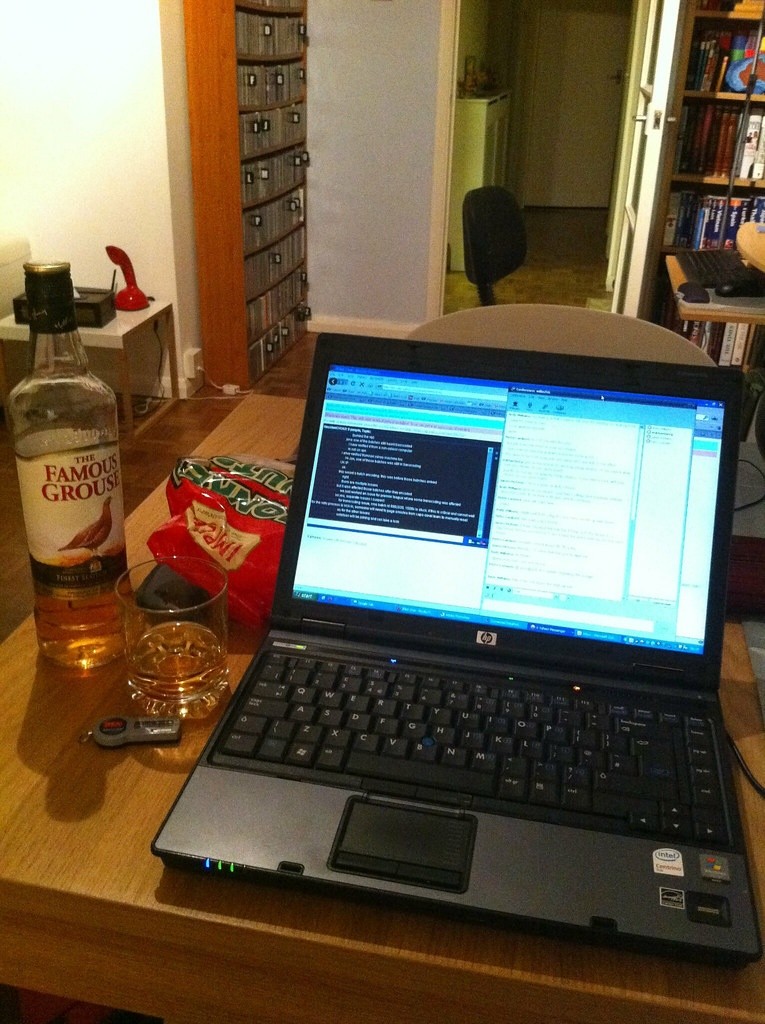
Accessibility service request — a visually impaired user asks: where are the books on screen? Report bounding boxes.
[236,60,305,104]
[661,183,765,252]
[685,0,765,95]
[234,11,306,55]
[238,102,306,156]
[241,182,305,250]
[241,141,306,204]
[651,282,765,377]
[244,224,305,298]
[247,262,306,345]
[248,298,305,384]
[672,99,765,181]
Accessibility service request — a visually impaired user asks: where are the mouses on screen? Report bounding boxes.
[715,281,748,297]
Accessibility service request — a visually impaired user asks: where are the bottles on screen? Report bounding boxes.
[7,258,143,669]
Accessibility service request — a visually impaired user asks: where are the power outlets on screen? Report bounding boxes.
[185,348,205,379]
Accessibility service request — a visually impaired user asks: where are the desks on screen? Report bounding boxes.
[667,255,765,324]
[0,300,180,446]
[1,393,765,1024]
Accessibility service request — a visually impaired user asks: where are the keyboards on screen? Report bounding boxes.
[676,249,747,289]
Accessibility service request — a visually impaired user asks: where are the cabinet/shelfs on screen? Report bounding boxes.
[184,0,309,391]
[636,0,765,443]
[450,90,513,272]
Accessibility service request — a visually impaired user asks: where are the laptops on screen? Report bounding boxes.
[148,332,765,973]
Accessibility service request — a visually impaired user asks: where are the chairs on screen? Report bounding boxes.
[403,305,718,369]
[463,186,528,306]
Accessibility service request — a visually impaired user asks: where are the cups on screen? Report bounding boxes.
[113,556,230,720]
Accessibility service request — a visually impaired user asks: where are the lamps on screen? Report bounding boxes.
[105,246,149,311]
